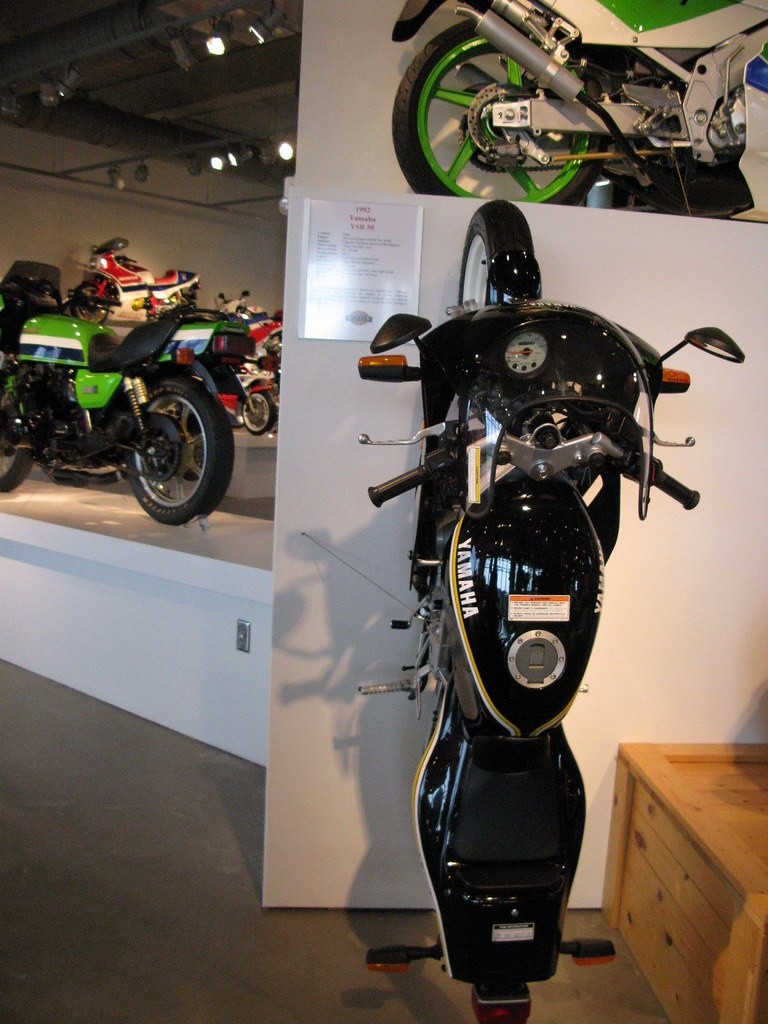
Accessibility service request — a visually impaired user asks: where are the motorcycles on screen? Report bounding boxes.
[359,199,745,1022]
[390,1,767,218]
[0,238,284,526]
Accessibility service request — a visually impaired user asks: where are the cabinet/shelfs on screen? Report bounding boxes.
[601,743,768,1024]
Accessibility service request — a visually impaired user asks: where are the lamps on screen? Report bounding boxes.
[206,19,232,56]
[248,3,287,44]
[165,26,198,69]
[211,149,253,170]
[38,69,75,106]
[254,138,277,164]
[183,147,201,175]
[135,155,150,183]
[107,162,125,191]
[0,85,24,123]
